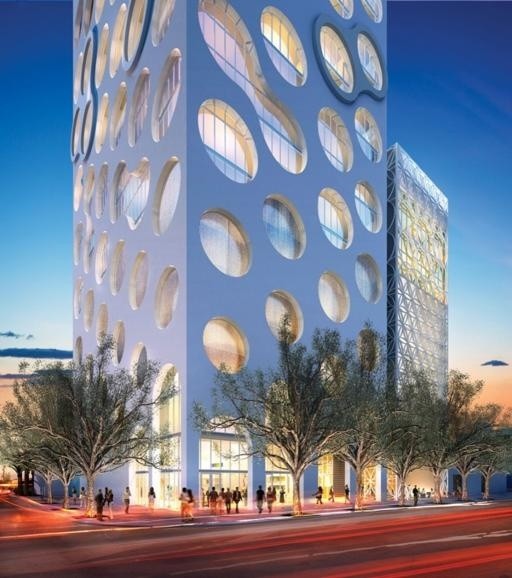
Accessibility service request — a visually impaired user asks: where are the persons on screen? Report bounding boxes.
[70,484,351,522]
[413,485,419,506]
